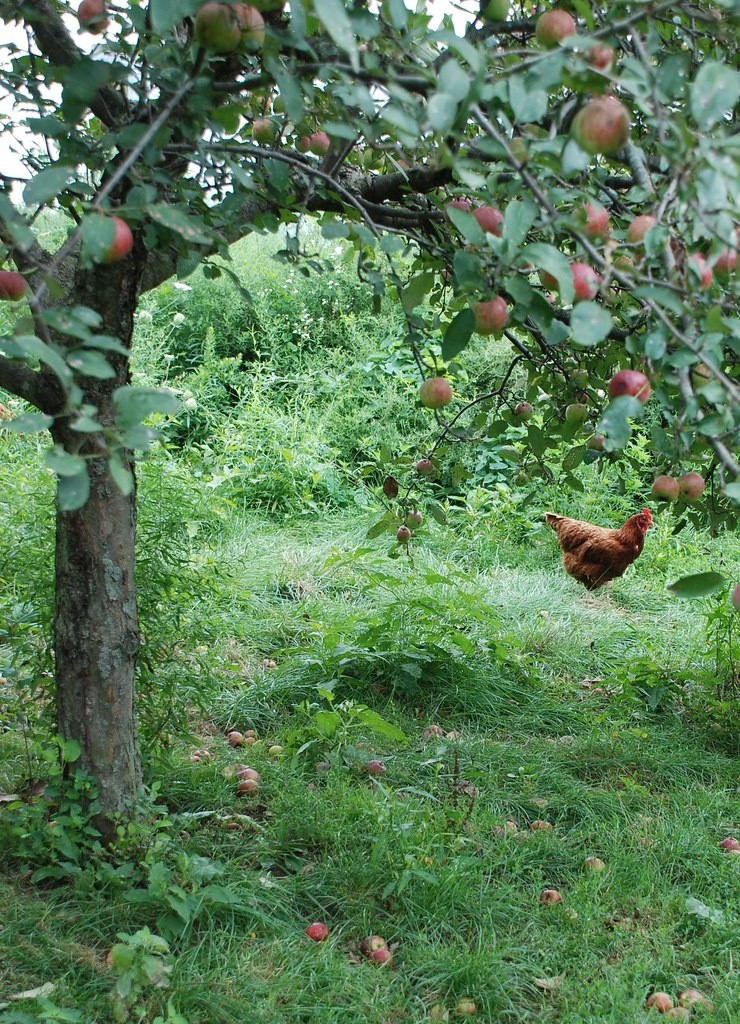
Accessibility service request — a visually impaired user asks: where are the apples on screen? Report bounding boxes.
[1,582,740,1019]
[0,0,740,541]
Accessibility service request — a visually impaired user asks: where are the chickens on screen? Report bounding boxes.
[543,507,654,594]
